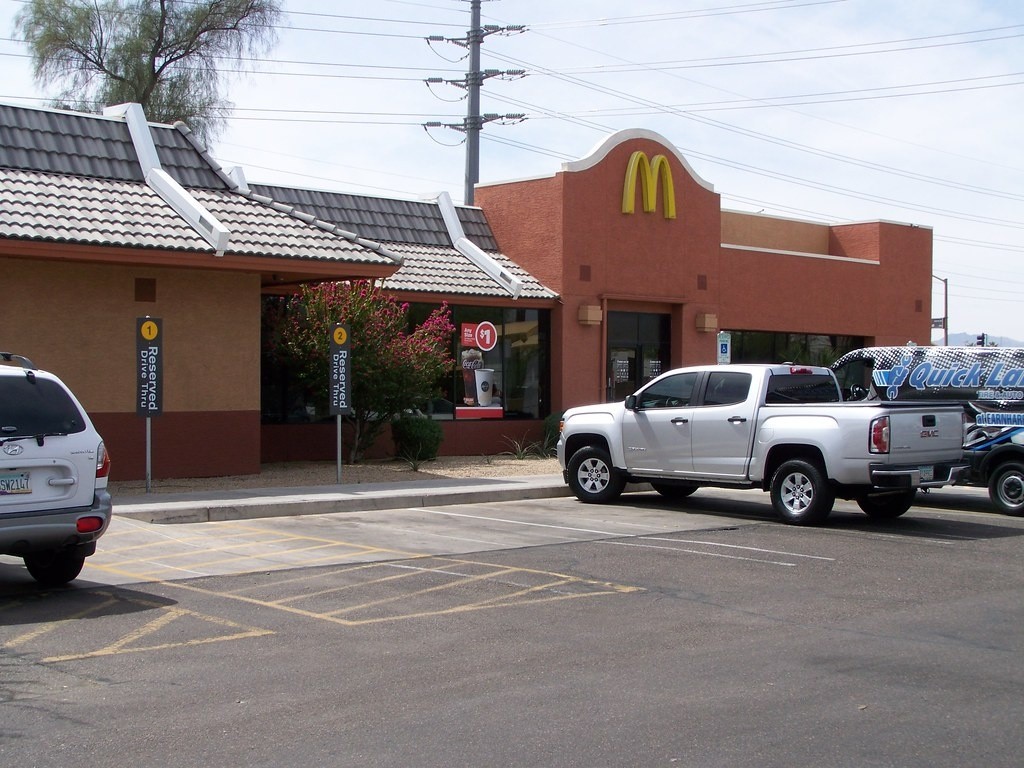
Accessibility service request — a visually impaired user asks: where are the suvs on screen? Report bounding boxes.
[0,351,112,589]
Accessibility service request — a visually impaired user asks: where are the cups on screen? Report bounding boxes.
[474,368,494,406]
[461,351,483,405]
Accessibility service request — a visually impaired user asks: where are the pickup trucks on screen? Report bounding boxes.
[556,363,973,527]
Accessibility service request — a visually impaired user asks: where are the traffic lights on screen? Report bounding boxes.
[977,333,985,347]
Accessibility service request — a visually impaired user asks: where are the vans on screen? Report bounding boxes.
[826,346,1024,515]
